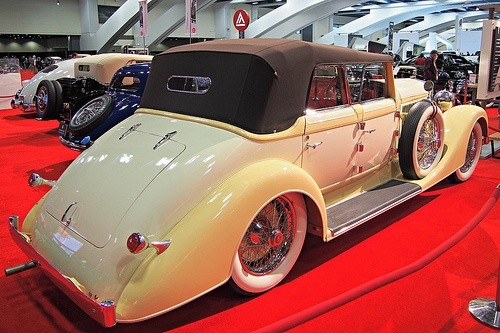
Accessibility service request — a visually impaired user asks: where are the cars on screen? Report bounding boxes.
[4,38,490,329]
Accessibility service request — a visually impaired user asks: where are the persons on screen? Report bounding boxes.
[2,53,52,73]
[414,53,425,65]
[72,53,77,59]
[424,50,439,95]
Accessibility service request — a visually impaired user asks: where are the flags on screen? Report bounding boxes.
[139,1,147,37]
[184,0,197,34]
